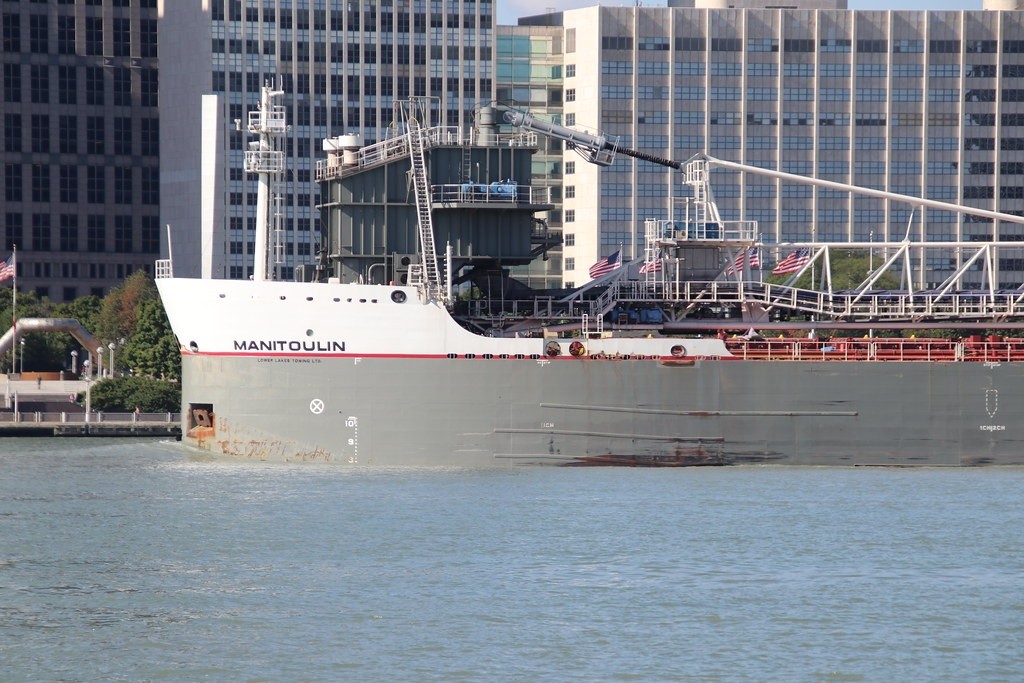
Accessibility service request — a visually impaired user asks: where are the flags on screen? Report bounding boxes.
[728,247,759,275]
[639,250,669,274]
[0,252,14,282]
[589,249,621,280]
[772,248,812,276]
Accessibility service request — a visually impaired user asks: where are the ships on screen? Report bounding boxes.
[152,75,1024,471]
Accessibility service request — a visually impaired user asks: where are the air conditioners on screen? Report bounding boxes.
[394,254,418,285]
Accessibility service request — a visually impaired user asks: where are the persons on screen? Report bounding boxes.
[36,374,42,390]
[717,329,727,341]
[135,406,140,421]
